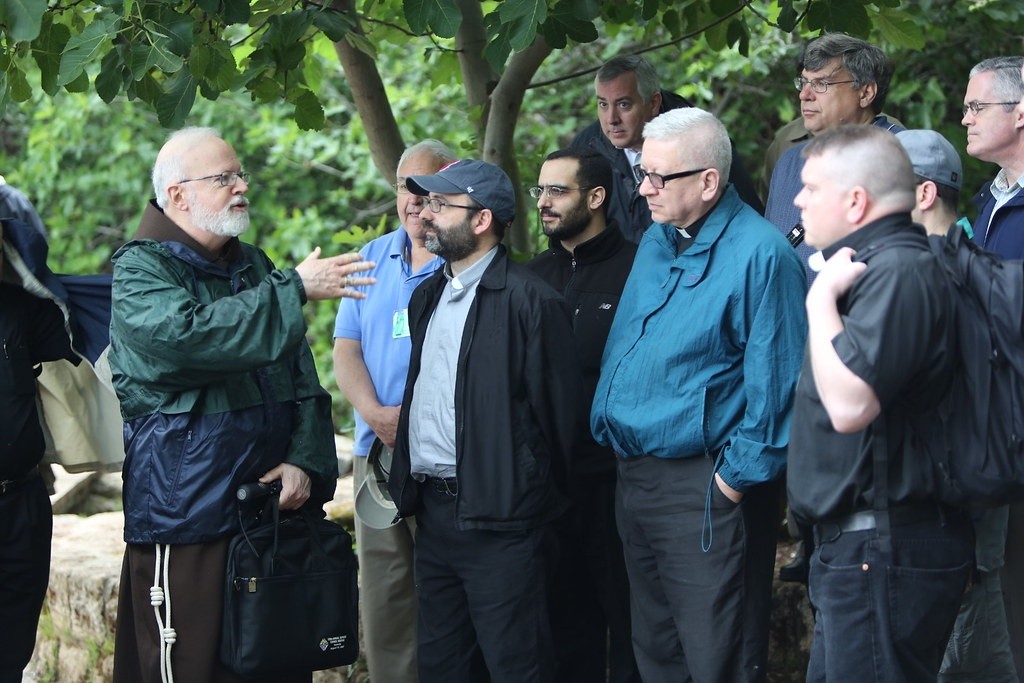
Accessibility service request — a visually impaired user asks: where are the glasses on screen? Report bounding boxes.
[177,173,249,187]
[421,196,497,221]
[392,181,410,195]
[793,75,864,93]
[529,185,598,199]
[963,101,1020,116]
[633,164,709,189]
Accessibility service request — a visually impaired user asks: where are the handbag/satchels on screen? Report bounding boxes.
[220,494,358,677]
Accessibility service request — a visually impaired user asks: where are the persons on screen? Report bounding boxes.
[106,126,377,683]
[895,129,1020,683]
[522,145,641,683]
[572,53,765,245]
[331,139,458,683]
[387,160,584,683]
[590,107,808,683]
[961,56,1024,262]
[786,124,975,683]
[0,218,53,683]
[767,32,908,290]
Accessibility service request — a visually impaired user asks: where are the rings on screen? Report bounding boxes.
[345,278,350,288]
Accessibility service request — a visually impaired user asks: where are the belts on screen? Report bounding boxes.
[816,500,951,544]
[420,478,458,495]
[0,463,39,498]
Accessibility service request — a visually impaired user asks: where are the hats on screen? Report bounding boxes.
[892,129,963,189]
[355,434,404,530]
[406,157,517,227]
